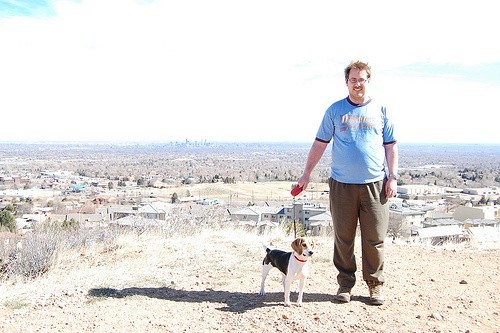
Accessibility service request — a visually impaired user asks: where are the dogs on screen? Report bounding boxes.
[259,237,316,307]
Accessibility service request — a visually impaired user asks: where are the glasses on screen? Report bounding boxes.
[348,77,368,84]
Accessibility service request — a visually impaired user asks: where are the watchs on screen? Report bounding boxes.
[388,174,398,180]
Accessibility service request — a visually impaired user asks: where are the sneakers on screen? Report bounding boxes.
[366,280,387,306]
[335,284,353,303]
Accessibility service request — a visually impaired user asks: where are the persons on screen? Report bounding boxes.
[299,61,397,306]
